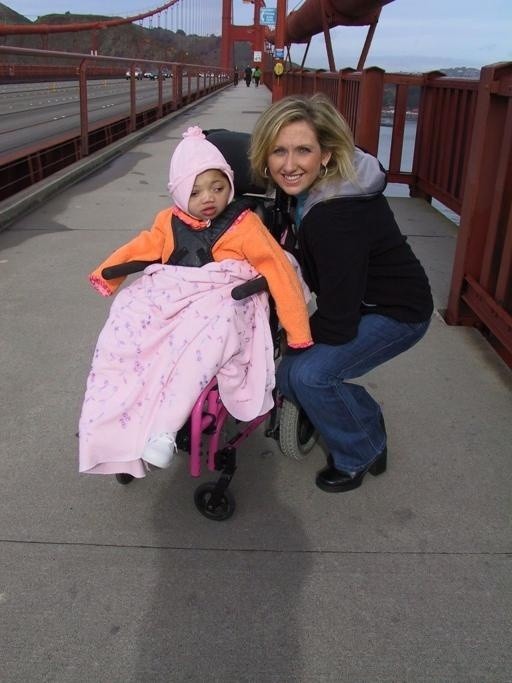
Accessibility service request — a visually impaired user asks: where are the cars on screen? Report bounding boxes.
[126,67,171,80]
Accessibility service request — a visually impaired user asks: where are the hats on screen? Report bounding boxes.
[166,126,236,221]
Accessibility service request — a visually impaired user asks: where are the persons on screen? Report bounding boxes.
[232,64,261,88]
[247,92,434,494]
[79,124,314,486]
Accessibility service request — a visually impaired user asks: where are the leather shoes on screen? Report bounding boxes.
[316,444,388,493]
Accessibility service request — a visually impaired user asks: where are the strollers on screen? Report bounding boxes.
[101,126,320,521]
